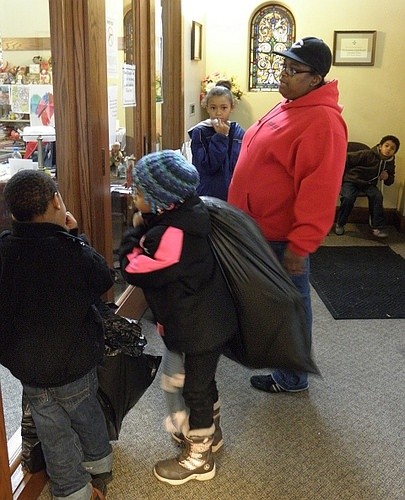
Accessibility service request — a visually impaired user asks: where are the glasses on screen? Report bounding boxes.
[280,63,314,76]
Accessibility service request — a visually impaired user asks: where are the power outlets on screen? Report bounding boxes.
[190,103,196,117]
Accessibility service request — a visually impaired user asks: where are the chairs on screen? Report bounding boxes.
[343,142,385,230]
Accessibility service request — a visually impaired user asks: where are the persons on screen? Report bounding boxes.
[188,80,246,201]
[334,135,400,238]
[120,150,237,486]
[0,167,118,500]
[229,36,352,392]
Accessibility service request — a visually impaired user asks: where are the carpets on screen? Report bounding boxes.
[306,246,405,320]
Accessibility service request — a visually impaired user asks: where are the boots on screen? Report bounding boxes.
[166,397,224,452]
[154,435,215,485]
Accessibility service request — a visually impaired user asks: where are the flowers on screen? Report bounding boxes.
[200,73,243,109]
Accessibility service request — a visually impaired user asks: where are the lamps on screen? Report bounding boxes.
[22,125,55,173]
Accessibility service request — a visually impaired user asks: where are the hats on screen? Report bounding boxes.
[272,37,332,78]
[131,151,199,214]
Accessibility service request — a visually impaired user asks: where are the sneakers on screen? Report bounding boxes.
[250,374,284,393]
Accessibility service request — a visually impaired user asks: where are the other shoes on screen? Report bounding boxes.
[367,229,389,240]
[336,225,344,234]
[90,470,113,500]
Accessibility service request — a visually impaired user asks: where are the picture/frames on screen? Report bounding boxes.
[191,21,202,61]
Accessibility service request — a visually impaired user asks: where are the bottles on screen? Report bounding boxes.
[11,147,22,177]
